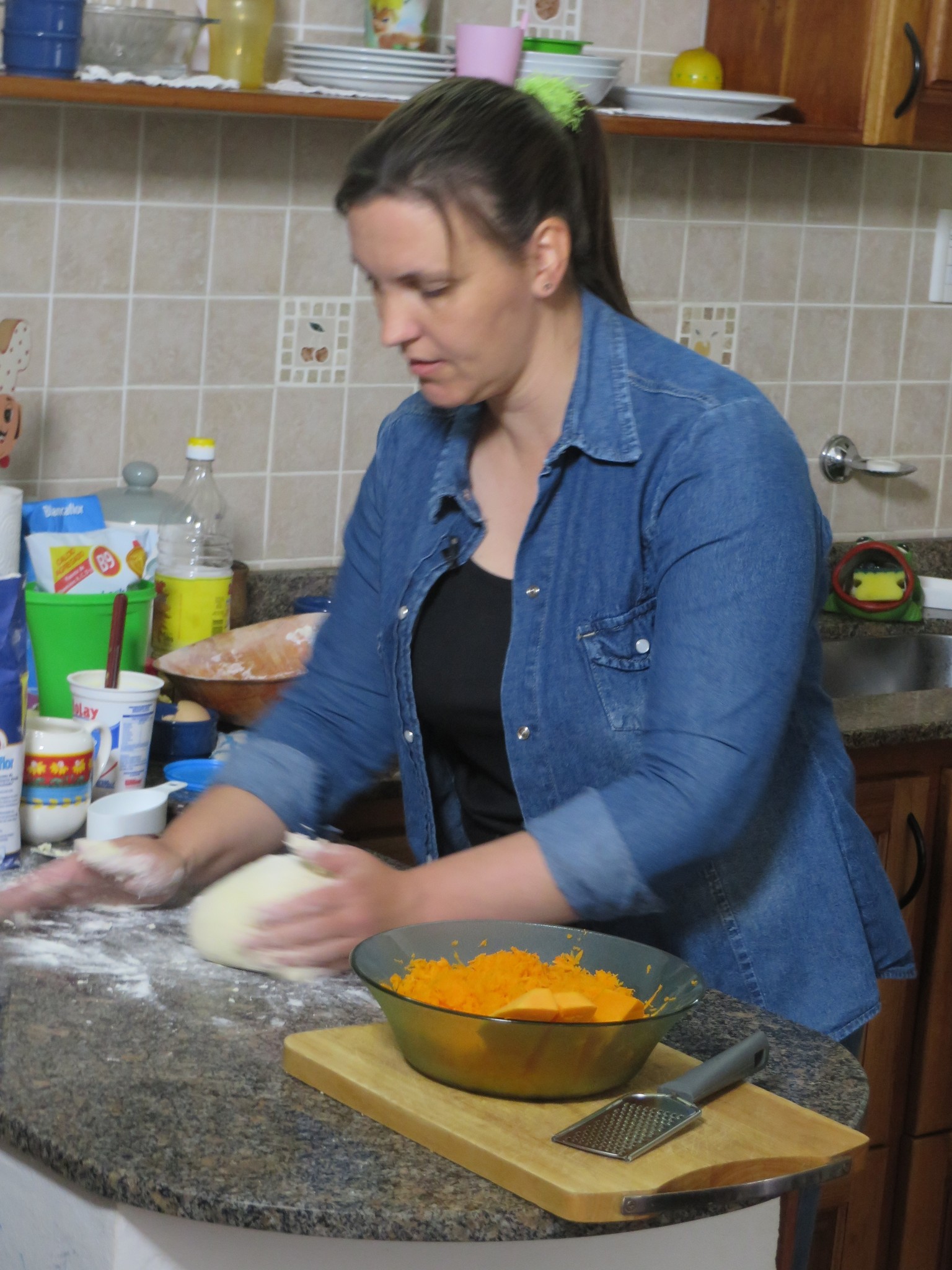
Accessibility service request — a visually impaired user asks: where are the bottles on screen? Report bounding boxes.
[90,461,202,572]
[148,435,235,660]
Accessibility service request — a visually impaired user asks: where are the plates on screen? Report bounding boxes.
[605,85,796,120]
[281,39,457,100]
[522,34,594,55]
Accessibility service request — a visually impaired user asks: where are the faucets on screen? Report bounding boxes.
[820,435,918,484]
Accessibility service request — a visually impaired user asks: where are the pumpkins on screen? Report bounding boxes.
[477,989,646,1073]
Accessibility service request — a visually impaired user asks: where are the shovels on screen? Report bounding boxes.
[547,1032,773,1163]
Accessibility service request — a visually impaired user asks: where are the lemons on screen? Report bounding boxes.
[671,46,723,91]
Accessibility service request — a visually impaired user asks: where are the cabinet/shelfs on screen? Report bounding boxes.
[782,738,952,1270]
[0,0,952,155]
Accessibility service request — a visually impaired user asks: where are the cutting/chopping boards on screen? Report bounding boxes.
[283,1023,870,1222]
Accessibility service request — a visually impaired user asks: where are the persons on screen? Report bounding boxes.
[0,76,918,1270]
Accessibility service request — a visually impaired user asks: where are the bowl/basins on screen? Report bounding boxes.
[350,918,703,1100]
[515,50,625,105]
[153,610,329,726]
[82,6,220,80]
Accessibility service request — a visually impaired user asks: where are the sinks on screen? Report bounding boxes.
[815,617,952,700]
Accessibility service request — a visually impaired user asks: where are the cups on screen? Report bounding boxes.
[3,0,87,80]
[66,670,164,802]
[365,0,432,50]
[23,580,158,719]
[294,597,333,612]
[152,701,219,763]
[230,560,248,628]
[206,1,275,90]
[17,715,112,846]
[162,758,225,813]
[458,13,529,87]
